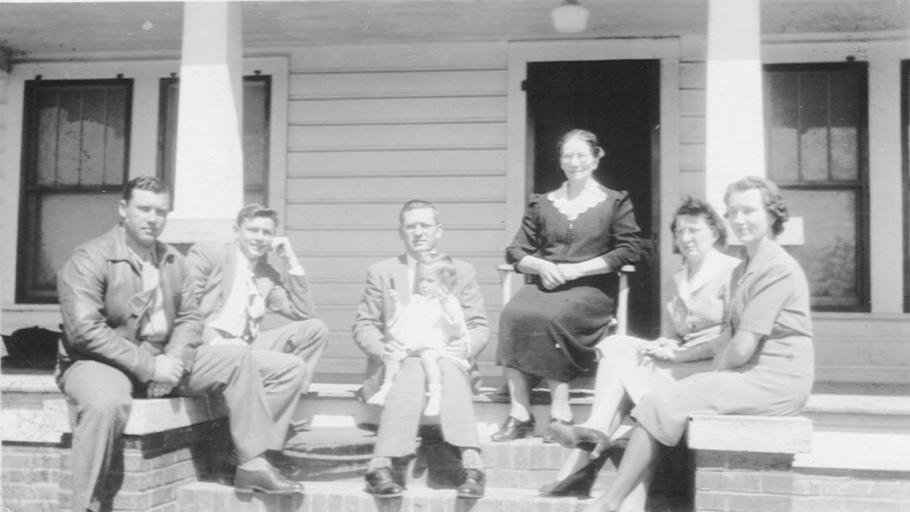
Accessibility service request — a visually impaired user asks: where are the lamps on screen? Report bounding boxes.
[548,0,591,34]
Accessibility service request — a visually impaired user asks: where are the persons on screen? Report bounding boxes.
[349,199,490,499]
[536,192,742,499]
[582,174,814,511]
[363,253,471,417]
[488,129,645,442]
[172,203,329,484]
[55,174,305,511]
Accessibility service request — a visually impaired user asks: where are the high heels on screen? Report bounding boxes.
[549,421,611,461]
[539,463,594,498]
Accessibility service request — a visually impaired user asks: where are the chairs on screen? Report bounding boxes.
[495,261,638,397]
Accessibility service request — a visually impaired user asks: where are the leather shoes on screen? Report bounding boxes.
[285,419,308,440]
[366,467,404,499]
[456,468,484,498]
[491,415,535,442]
[543,415,575,442]
[234,465,304,495]
